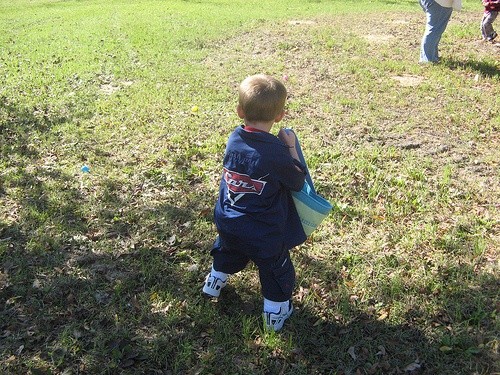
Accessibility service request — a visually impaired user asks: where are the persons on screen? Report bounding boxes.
[419,0,461,66]
[203,74,304,331]
[480,0,500,42]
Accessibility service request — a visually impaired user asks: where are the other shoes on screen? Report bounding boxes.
[483,32,498,42]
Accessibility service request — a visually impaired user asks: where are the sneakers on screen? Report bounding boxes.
[202,271,231,297]
[262,300,293,332]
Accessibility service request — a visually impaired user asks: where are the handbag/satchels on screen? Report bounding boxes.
[279,128,333,237]
